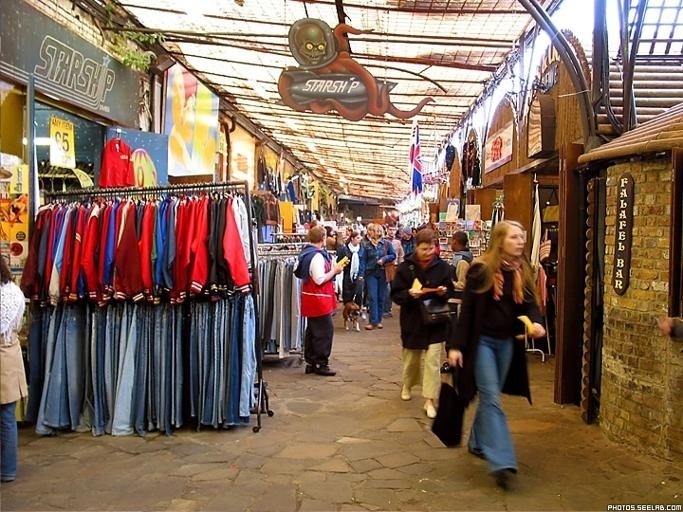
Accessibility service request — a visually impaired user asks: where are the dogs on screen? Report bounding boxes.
[342,300,366,332]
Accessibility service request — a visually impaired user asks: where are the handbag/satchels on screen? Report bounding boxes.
[542,190,560,222]
[539,228,552,262]
[431,364,463,447]
[419,297,452,327]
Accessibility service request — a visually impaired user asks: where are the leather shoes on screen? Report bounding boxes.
[312,364,336,376]
[468,447,486,460]
[423,402,437,419]
[401,383,411,401]
[305,365,314,374]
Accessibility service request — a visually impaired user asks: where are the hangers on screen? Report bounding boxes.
[35,181,246,218]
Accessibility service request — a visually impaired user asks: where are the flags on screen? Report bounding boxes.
[408,121,422,196]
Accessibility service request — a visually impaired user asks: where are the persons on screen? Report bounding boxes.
[12,194,25,223]
[294,219,441,377]
[388,229,454,418]
[446,219,546,491]
[658,317,683,341]
[0,253,30,483]
[441,232,474,374]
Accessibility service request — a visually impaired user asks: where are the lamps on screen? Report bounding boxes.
[148,51,177,76]
